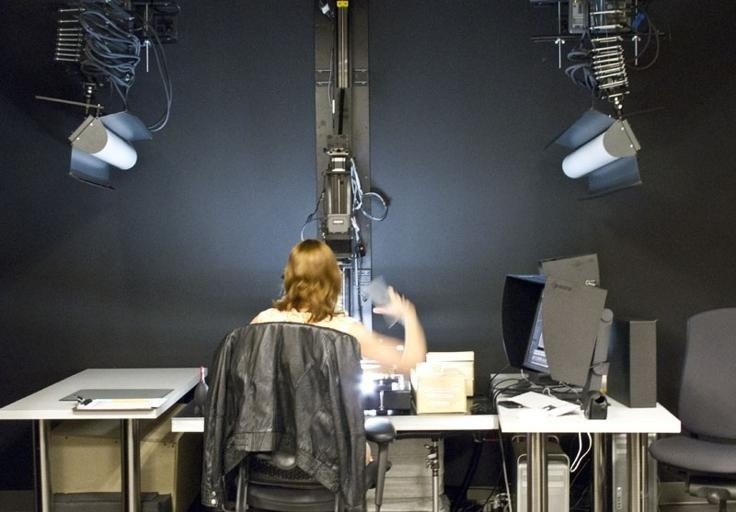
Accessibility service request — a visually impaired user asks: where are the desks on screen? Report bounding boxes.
[487,368,681,512]
[0,368,208,511]
[167,413,504,512]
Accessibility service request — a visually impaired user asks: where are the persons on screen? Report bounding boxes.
[250,238,427,466]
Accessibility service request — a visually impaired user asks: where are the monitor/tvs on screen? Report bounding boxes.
[524,288,553,375]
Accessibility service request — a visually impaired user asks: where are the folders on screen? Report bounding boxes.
[70,396,155,414]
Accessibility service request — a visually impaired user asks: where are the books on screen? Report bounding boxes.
[73,395,167,411]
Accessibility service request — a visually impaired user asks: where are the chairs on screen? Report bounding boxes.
[647,303,735,510]
[207,324,393,512]
